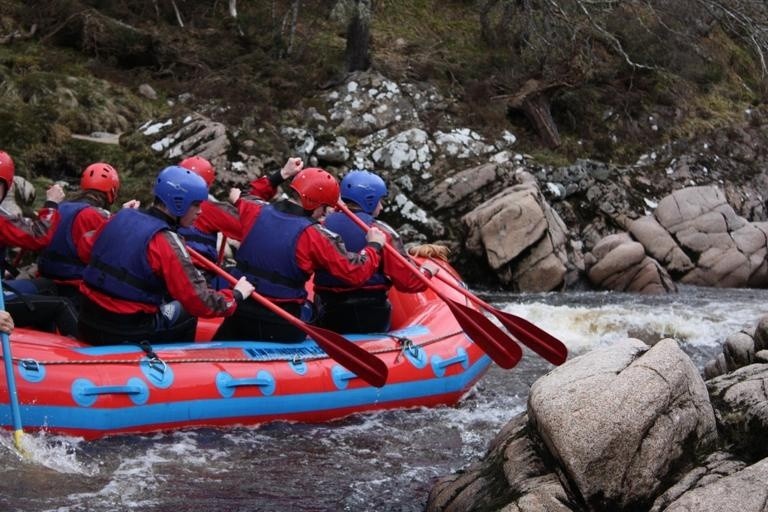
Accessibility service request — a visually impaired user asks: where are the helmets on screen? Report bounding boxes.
[153,164,209,217]
[176,154,217,190]
[79,161,121,205]
[340,168,389,215]
[0,150,16,194]
[288,166,341,211]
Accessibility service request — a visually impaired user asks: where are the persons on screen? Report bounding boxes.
[0,279,83,336]
[36,163,139,291]
[210,159,387,343]
[312,171,440,334]
[77,166,254,344]
[178,157,259,262]
[0,153,66,262]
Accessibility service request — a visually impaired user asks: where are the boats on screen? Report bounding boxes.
[0,255,492,440]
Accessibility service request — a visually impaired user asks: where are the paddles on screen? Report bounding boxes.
[184,243,388,387]
[338,202,523,368]
[409,254,569,365]
